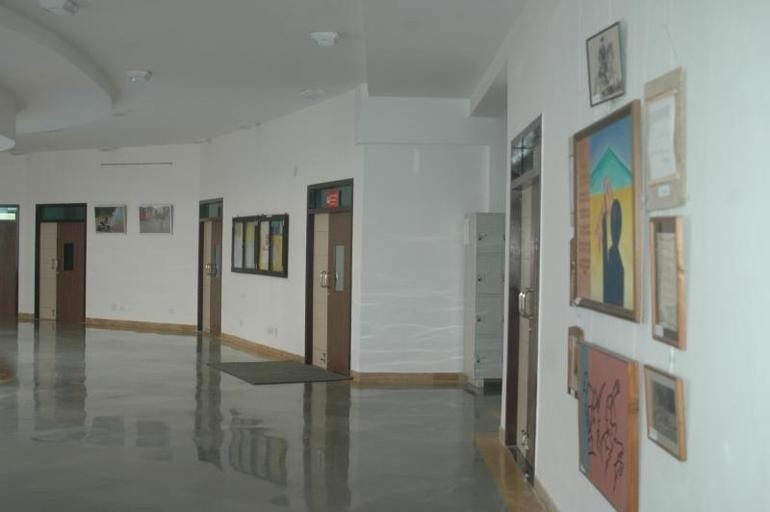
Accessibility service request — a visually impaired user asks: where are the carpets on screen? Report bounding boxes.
[207,361,354,385]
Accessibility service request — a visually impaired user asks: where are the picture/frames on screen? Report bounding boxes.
[568,326,583,400]
[93,205,127,235]
[576,341,639,512]
[649,217,687,351]
[586,23,625,107]
[138,204,173,236]
[569,99,643,324]
[644,366,687,461]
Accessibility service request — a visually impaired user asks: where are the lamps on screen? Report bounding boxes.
[39,0,78,17]
[311,32,340,47]
[128,70,151,83]
